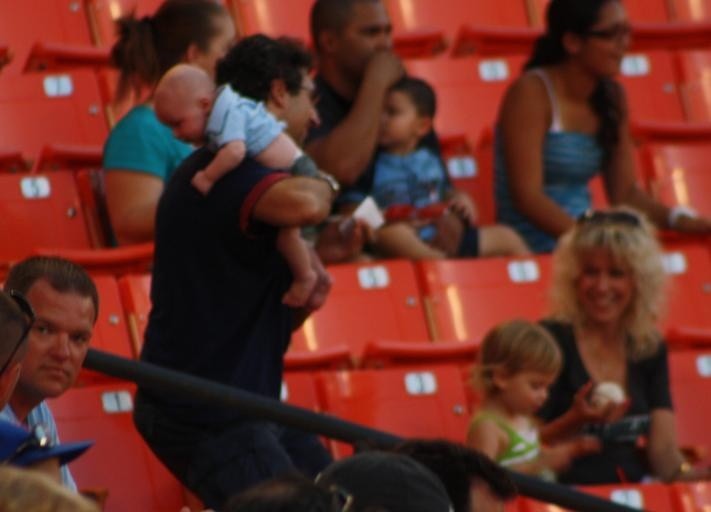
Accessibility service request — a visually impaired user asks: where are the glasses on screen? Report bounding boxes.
[288,84,320,99]
[9,423,52,463]
[586,19,631,42]
[579,212,644,225]
[1,285,36,378]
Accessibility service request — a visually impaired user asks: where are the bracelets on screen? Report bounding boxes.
[666,205,696,230]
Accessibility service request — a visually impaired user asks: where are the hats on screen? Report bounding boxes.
[0,419,96,467]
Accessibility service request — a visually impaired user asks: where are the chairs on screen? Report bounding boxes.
[0,0,711,511]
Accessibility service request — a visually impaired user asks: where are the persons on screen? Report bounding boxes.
[391,439,473,511]
[463,317,602,492]
[153,62,334,314]
[299,0,538,260]
[492,0,711,257]
[340,75,481,261]
[1,282,103,512]
[525,205,711,486]
[102,0,238,246]
[314,450,454,512]
[215,469,335,511]
[128,33,341,512]
[1,254,99,512]
